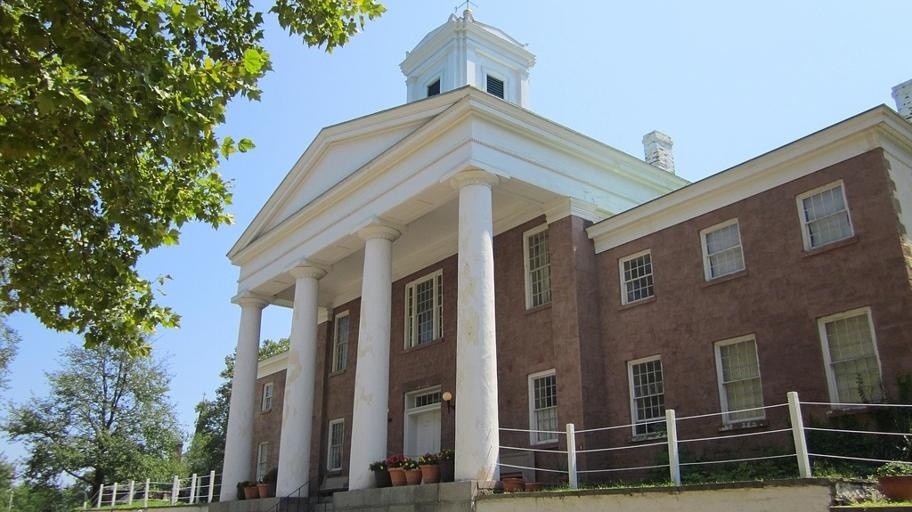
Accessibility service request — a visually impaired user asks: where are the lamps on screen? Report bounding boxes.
[388,408,392,422]
[442,391,455,414]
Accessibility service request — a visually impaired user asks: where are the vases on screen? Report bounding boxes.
[525,483,540,492]
[369,449,454,488]
[502,477,525,492]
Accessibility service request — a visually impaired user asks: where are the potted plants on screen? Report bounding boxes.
[237,464,278,500]
[876,461,912,502]
